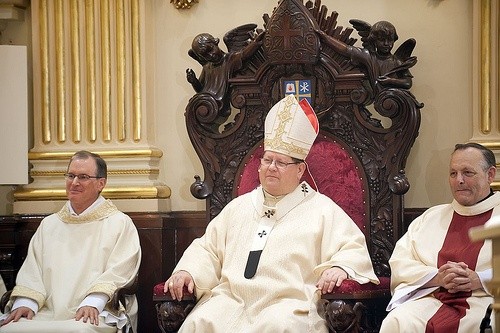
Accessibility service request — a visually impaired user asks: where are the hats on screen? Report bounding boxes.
[264,93,320,161]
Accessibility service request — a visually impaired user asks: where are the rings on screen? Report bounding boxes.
[453,283,455,288]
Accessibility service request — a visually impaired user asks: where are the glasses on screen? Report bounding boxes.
[63,173,100,182]
[260,158,297,169]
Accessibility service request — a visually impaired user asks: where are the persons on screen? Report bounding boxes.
[164,94,381,333]
[379,143,500,333]
[0,150,141,333]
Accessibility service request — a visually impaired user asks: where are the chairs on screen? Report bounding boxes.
[0,269,141,333]
[154,0,423,333]
